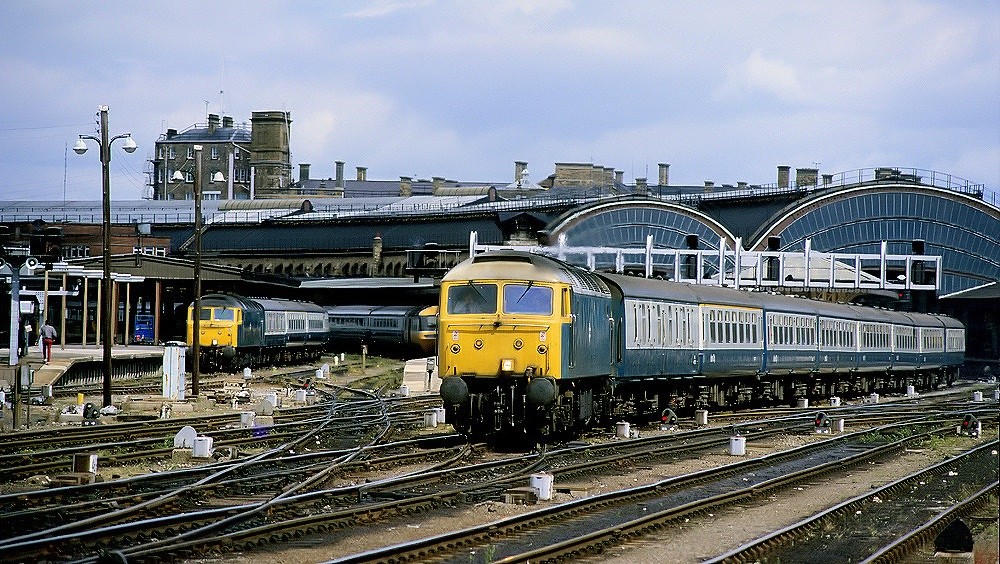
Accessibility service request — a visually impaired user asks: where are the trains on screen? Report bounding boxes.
[187,293,330,369]
[436,252,968,440]
[326,305,440,356]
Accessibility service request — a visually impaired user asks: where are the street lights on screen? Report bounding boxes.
[73,105,138,414]
[171,146,227,396]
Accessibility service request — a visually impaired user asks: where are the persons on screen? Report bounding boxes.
[41,320,57,363]
